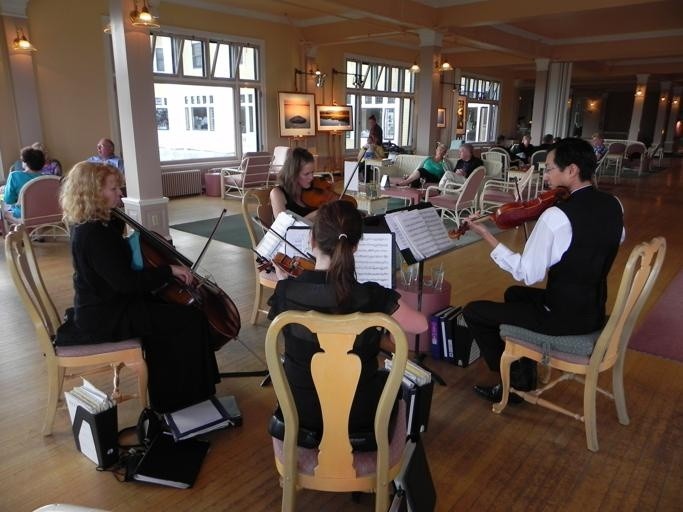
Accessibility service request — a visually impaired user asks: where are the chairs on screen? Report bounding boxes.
[0,160,71,243]
[205,141,667,230]
[4,224,148,437]
[264,311,408,512]
[241,186,288,325]
[493,236,667,453]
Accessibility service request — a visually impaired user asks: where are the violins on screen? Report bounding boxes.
[301,176,357,207]
[448,187,570,240]
[272,252,315,276]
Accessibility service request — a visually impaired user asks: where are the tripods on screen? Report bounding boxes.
[412,270,448,387]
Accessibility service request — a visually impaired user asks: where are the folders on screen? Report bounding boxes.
[430,305,462,363]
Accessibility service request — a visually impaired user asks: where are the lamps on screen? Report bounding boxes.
[434,53,454,72]
[330,69,364,85]
[587,83,679,107]
[7,22,39,55]
[408,53,424,75]
[126,0,159,29]
[291,66,327,87]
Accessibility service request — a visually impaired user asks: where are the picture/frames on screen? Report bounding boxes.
[457,95,468,136]
[274,90,317,140]
[434,108,447,128]
[313,103,353,133]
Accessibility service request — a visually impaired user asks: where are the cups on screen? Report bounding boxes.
[399,248,446,291]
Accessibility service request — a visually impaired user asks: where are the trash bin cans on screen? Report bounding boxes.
[205,173,220,196]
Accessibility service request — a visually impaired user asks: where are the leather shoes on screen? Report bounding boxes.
[471,380,526,408]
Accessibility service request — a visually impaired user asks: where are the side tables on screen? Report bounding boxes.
[389,274,450,355]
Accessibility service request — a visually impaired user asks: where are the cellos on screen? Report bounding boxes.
[111,207,241,347]
